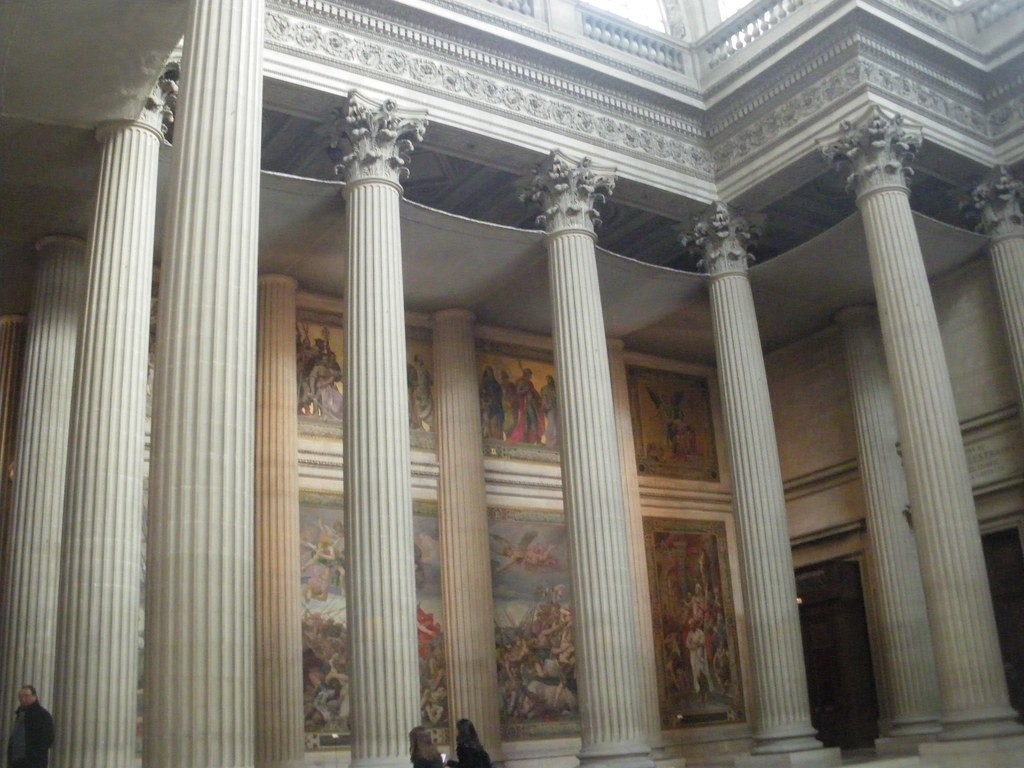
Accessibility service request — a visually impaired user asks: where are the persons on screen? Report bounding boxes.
[445,718,491,768]
[7,685,54,768]
[408,726,443,768]
